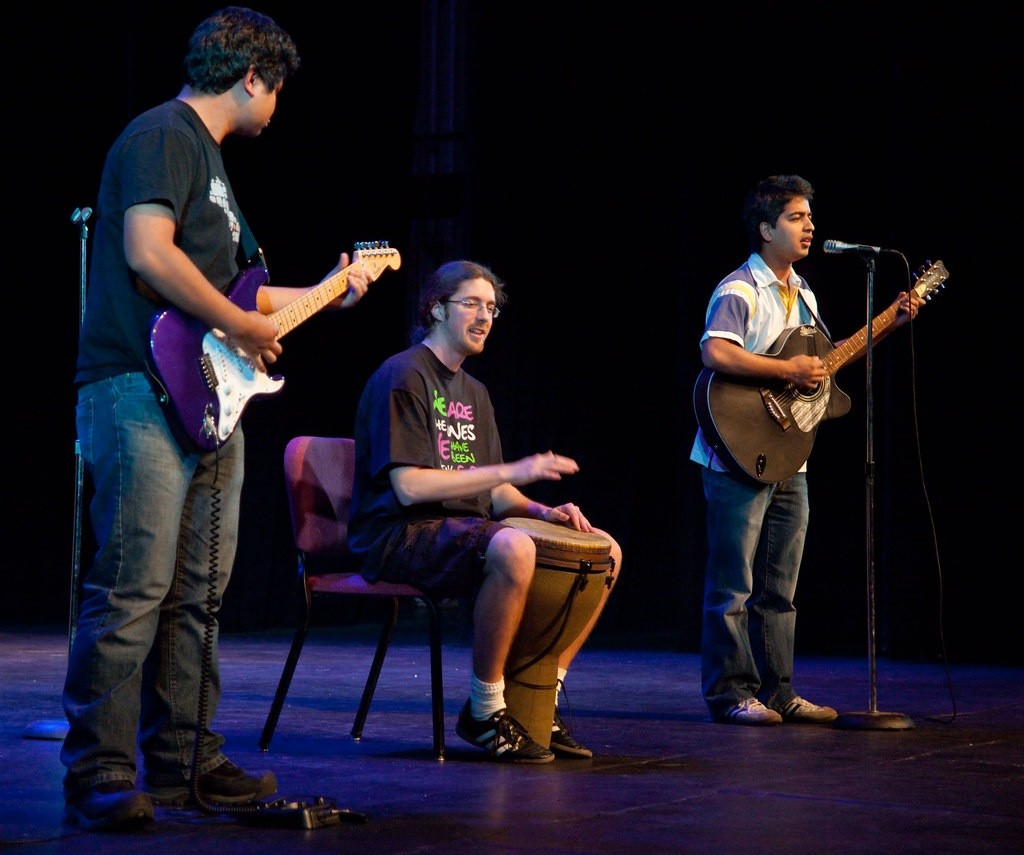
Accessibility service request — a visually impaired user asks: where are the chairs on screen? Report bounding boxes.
[258,435,471,768]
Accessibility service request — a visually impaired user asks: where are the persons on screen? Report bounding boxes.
[346,261,620,766]
[688,174,926,724]
[60,5,373,833]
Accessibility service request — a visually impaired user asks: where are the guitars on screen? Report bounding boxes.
[693,259,949,485]
[148,240,402,455]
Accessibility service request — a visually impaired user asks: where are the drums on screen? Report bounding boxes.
[501,516,612,749]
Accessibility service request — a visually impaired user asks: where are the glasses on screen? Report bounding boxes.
[440,297,501,318]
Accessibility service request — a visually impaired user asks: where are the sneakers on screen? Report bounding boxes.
[63,770,154,825]
[774,696,838,721]
[149,759,277,805]
[717,696,783,725]
[454,696,555,764]
[548,709,592,759]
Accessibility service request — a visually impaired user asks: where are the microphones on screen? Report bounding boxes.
[823,239,898,254]
